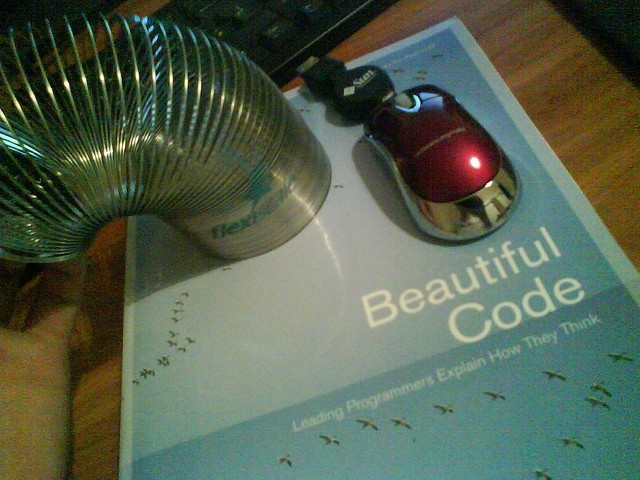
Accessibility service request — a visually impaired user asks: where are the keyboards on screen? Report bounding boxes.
[145,1,395,89]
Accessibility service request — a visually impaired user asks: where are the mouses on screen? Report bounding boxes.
[363,83,520,242]
